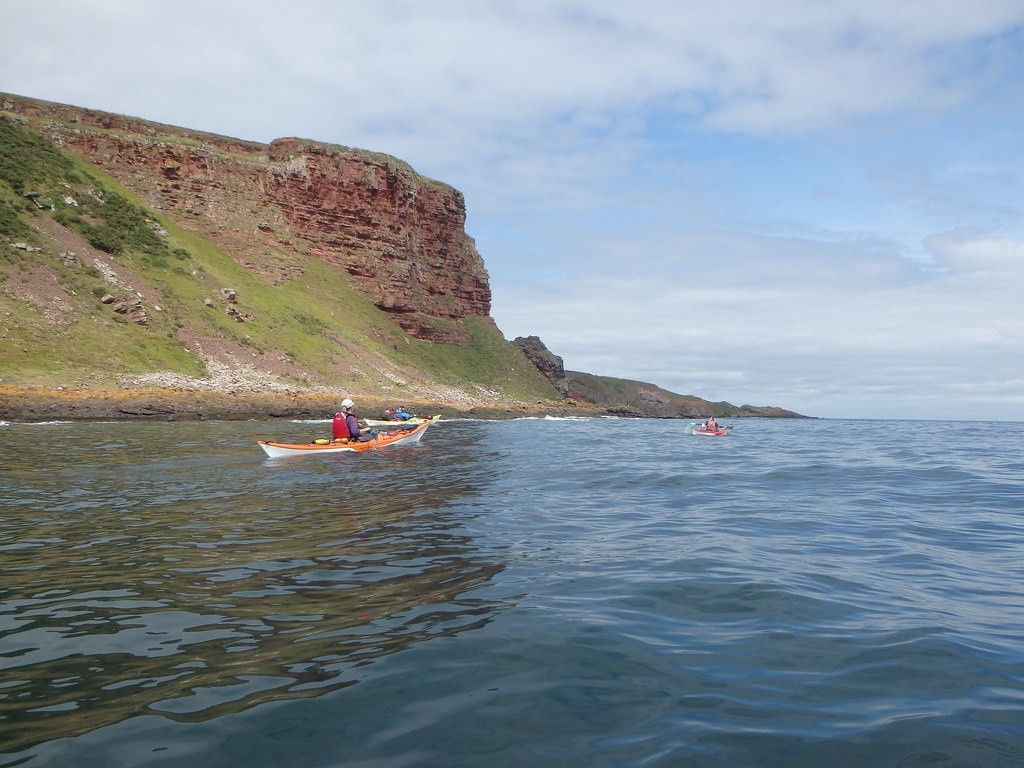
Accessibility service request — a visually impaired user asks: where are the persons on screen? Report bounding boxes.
[394,405,404,418]
[704,416,718,431]
[332,399,371,442]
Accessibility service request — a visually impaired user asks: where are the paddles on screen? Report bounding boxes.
[335,412,379,441]
[687,420,733,430]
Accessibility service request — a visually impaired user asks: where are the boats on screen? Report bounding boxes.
[690,427,728,437]
[363,415,443,426]
[255,418,431,458]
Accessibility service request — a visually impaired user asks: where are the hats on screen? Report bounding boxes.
[341,399,355,408]
